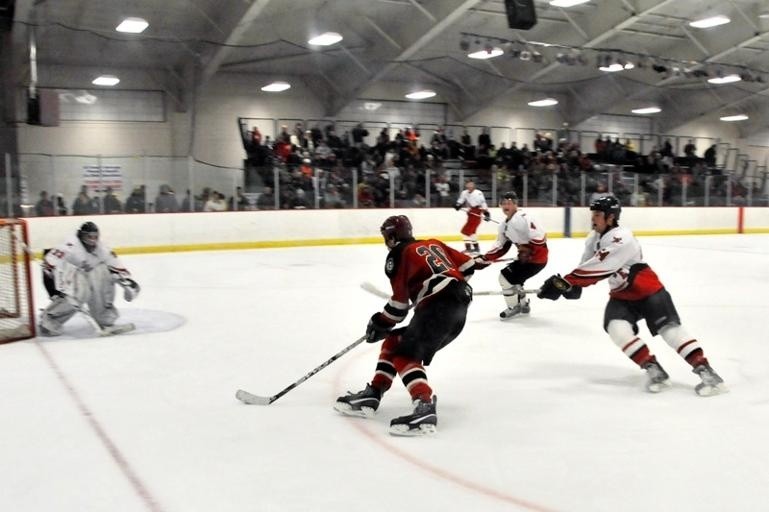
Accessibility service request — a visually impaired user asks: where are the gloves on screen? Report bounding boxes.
[454,203,461,211]
[365,312,396,343]
[537,274,569,301]
[562,284,583,300]
[483,211,490,221]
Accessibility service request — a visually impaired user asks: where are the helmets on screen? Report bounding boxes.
[499,191,518,205]
[589,193,621,222]
[76,221,99,252]
[379,214,413,242]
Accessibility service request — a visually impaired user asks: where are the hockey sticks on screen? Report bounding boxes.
[234,331,372,409]
[356,280,547,303]
[9,230,136,339]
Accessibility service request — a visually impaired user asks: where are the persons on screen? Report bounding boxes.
[333,215,476,438]
[456,180,492,254]
[650,138,760,206]
[39,222,137,335]
[473,192,549,320]
[459,124,649,206]
[276,152,459,210]
[35,183,275,216]
[249,122,457,152]
[538,195,728,396]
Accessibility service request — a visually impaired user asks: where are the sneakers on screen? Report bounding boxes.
[499,302,521,318]
[643,358,670,392]
[521,303,530,314]
[692,358,728,395]
[471,248,480,252]
[336,382,383,412]
[100,322,136,334]
[390,395,437,429]
[40,310,61,334]
[461,249,470,253]
